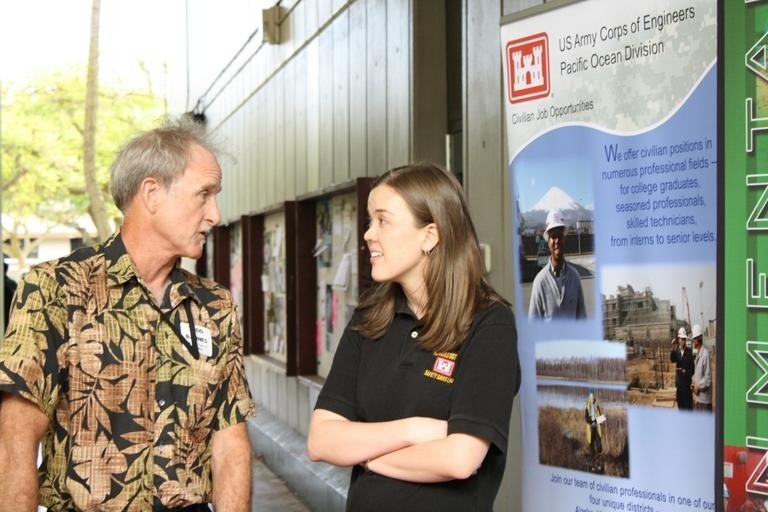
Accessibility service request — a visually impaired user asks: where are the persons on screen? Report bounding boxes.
[671,327,694,409]
[0,122,257,512]
[4,262,18,330]
[582,390,607,473]
[685,326,714,411]
[527,209,589,321]
[307,160,522,512]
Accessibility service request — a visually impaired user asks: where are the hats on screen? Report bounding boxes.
[677,326,688,340]
[541,209,568,243]
[691,324,704,339]
